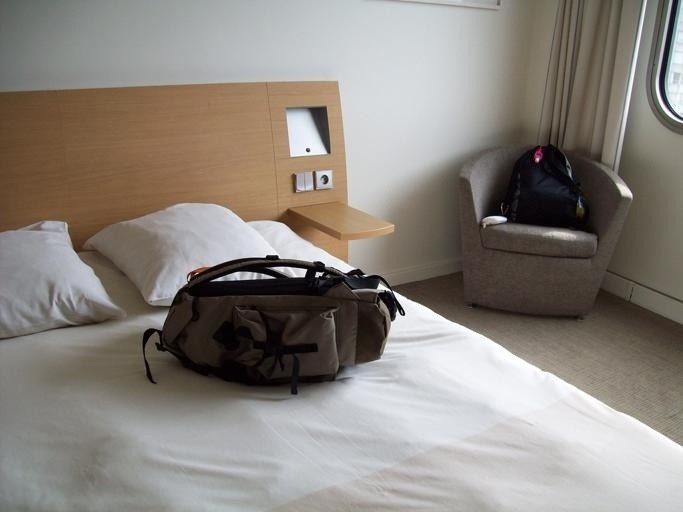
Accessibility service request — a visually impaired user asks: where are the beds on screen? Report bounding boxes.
[2,80,683,512]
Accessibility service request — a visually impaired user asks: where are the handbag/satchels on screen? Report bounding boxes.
[142,254,406,396]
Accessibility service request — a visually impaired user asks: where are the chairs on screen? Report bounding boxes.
[458,143,633,317]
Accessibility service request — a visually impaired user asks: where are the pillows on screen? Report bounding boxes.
[81,202,296,307]
[0,219,127,340]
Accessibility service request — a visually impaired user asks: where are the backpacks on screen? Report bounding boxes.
[500,144,590,229]
